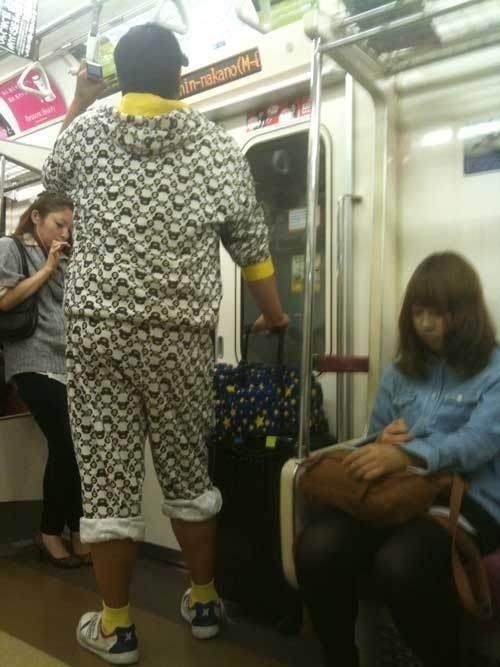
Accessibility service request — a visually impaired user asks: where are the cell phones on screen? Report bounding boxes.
[86,61,103,84]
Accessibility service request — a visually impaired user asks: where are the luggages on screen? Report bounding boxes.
[208,322,338,635]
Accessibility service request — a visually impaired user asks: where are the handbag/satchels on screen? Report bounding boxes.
[213,360,330,440]
[298,446,439,529]
[0,300,38,342]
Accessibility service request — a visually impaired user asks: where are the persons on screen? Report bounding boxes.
[40,21,291,665]
[0,189,94,570]
[292,249,500,666]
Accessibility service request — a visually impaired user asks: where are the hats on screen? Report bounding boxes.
[113,23,189,67]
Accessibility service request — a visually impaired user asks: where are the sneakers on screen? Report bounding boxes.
[76,611,141,664]
[180,587,222,639]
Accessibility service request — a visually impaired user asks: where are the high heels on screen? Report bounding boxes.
[39,531,93,569]
